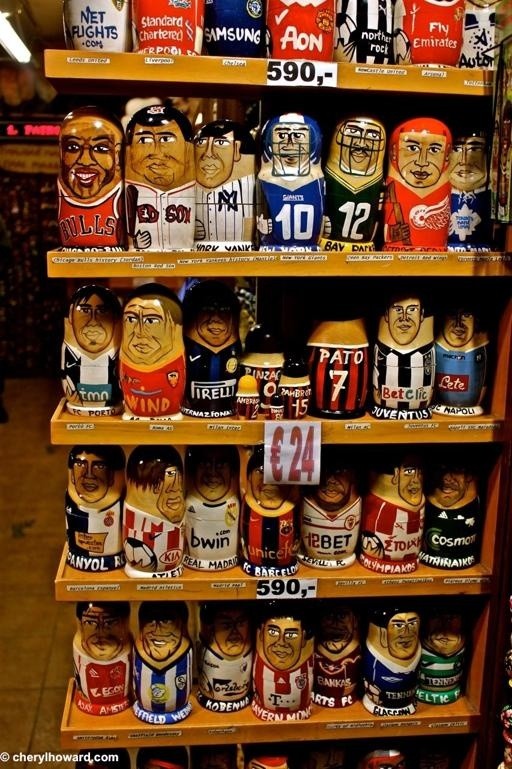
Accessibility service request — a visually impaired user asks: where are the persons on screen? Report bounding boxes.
[53,102,492,250]
[65,0,502,70]
[57,279,488,418]
[69,601,467,725]
[61,444,484,577]
[58,279,488,419]
[73,741,455,768]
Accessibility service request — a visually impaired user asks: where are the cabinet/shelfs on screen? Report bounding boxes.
[44,47,510,750]
